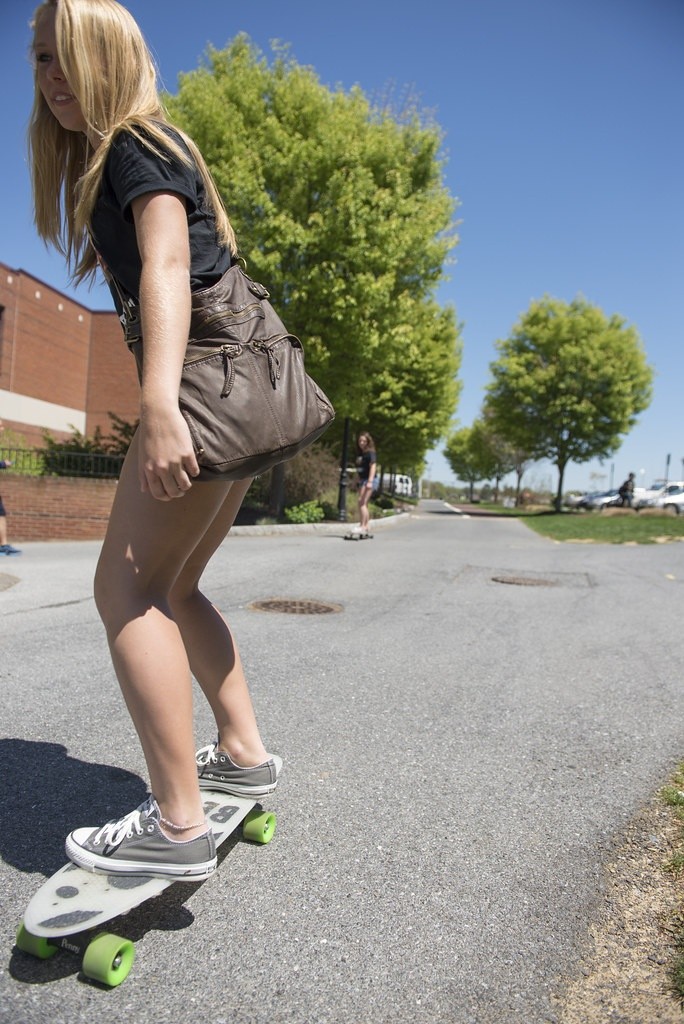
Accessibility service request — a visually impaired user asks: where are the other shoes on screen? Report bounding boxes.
[350,527,368,534]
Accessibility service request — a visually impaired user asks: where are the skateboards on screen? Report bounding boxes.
[21,746,288,989]
[342,530,374,541]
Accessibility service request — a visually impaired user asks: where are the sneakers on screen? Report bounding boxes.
[65,794,218,881]
[195,742,276,800]
[0,544,22,556]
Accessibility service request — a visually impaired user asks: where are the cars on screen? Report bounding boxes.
[552,479,684,517]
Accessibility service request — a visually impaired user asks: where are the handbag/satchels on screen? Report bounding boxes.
[124,264,335,480]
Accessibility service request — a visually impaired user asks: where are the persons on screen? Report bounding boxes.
[350,430,379,535]
[24,1,279,882]
[621,473,635,507]
[0,459,22,556]
